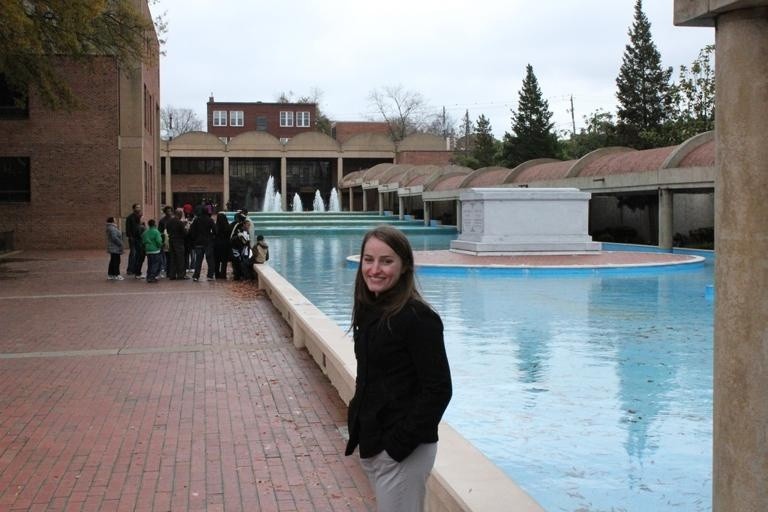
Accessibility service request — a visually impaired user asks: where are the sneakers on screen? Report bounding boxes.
[107,274,124,280]
[207,272,227,281]
[127,269,198,282]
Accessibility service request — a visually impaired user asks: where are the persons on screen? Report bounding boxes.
[105,199,269,282]
[345,224,453,511]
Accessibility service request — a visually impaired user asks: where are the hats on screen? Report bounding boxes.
[184,204,193,213]
